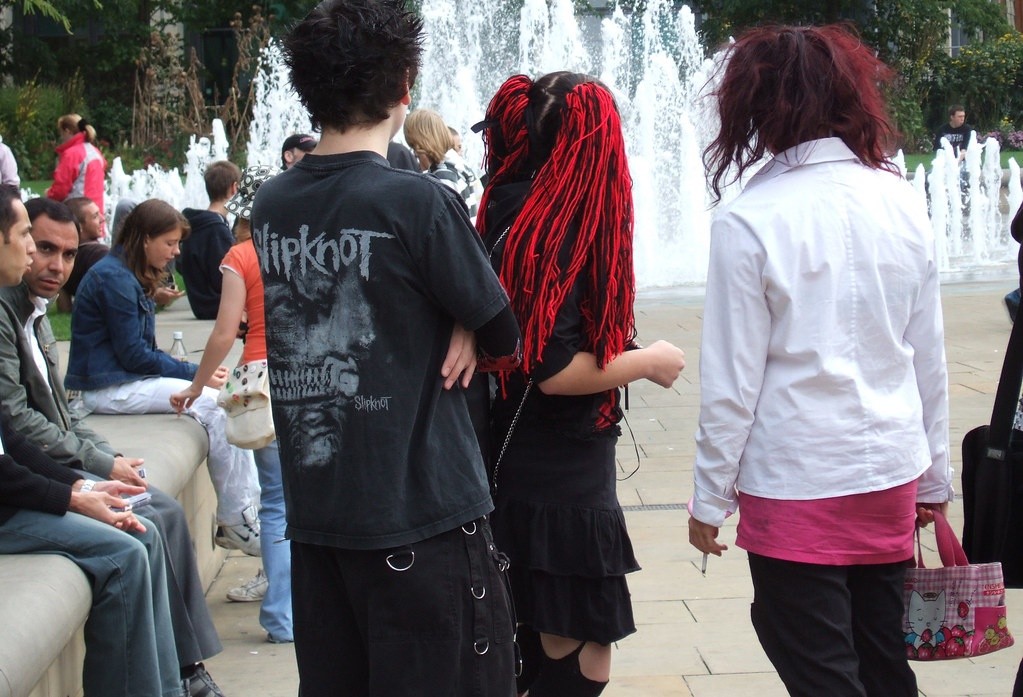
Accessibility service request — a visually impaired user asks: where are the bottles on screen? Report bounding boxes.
[168,332,188,361]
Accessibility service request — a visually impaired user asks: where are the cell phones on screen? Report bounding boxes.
[111,492,151,513]
[137,469,146,479]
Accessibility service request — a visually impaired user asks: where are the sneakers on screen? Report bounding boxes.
[215,508,262,556]
[185,661,225,697]
[225,569,270,601]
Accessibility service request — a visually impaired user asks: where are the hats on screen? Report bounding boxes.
[281,134,318,153]
[224,166,278,220]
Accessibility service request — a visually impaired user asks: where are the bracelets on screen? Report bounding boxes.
[80,479,94,492]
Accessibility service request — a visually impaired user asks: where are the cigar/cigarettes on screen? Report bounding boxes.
[702,553,707,574]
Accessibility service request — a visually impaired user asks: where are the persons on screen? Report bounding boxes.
[280,133,318,171]
[175,160,241,320]
[58,198,182,314]
[0,182,224,697]
[0,136,20,186]
[933,105,970,155]
[171,219,293,643]
[387,109,478,226]
[45,113,105,216]
[250,0,523,697]
[687,23,955,697]
[471,69,685,697]
[65,197,262,559]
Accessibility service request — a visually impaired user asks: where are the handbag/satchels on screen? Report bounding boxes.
[217,358,276,451]
[902,510,1015,661]
[962,424,1023,589]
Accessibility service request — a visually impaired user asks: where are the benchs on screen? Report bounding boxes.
[0,321,247,697]
